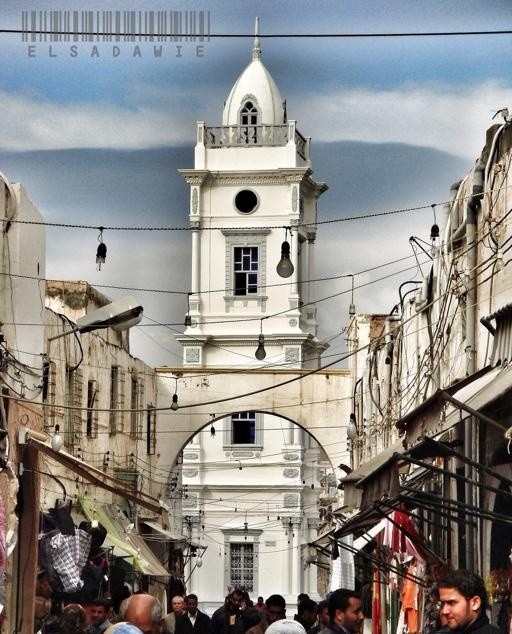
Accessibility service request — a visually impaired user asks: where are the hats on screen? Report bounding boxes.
[264,619,306,634]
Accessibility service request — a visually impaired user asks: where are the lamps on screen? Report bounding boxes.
[255,320,266,361]
[171,377,179,411]
[184,293,191,326]
[349,275,355,314]
[96,229,107,272]
[196,551,202,567]
[430,205,440,240]
[52,424,63,453]
[347,414,358,440]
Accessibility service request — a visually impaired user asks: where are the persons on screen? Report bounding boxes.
[35,567,512,634]
